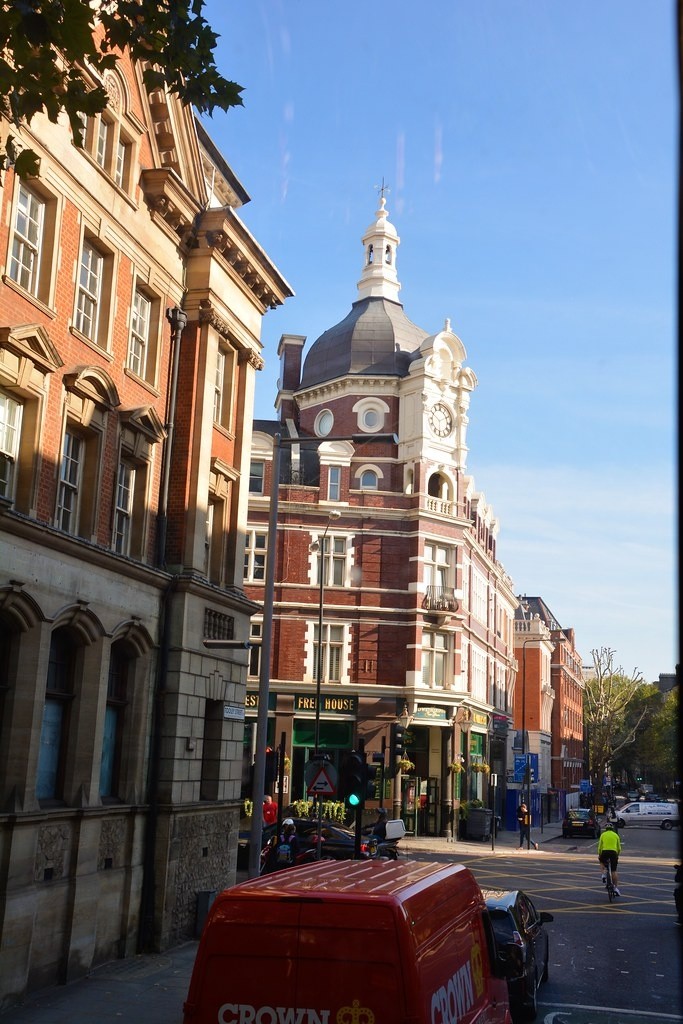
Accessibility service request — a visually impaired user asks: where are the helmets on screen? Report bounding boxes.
[605,823,614,829]
[376,808,388,818]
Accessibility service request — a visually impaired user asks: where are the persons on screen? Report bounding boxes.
[520,904,531,928]
[364,807,387,843]
[262,818,325,876]
[579,782,632,811]
[262,796,277,826]
[518,804,538,849]
[598,823,622,897]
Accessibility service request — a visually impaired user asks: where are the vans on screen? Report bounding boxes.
[182,856,513,1024]
[607,802,680,831]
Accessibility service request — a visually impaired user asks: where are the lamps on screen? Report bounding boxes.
[457,710,471,734]
[397,704,410,730]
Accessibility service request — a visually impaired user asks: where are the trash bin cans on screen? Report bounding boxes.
[494,816,502,838]
[466,808,493,842]
[616,796,626,808]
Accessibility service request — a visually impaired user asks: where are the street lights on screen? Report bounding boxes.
[248,433,401,880]
[308,509,343,816]
[522,637,567,851]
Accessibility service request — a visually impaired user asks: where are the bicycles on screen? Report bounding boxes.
[605,859,619,904]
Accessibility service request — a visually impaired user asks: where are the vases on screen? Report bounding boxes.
[452,768,461,772]
[401,765,410,771]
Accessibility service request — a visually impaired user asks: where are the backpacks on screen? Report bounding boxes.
[277,835,294,866]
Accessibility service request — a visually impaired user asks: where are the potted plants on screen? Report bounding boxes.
[243,798,253,818]
[472,762,490,776]
[459,799,483,820]
[290,799,347,825]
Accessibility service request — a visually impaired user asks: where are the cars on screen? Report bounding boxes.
[625,783,661,804]
[238,817,379,869]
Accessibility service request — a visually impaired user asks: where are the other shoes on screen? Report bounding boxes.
[614,887,621,897]
[601,874,607,884]
[517,847,524,851]
[535,843,538,850]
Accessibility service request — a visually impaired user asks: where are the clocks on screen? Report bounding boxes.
[429,404,453,436]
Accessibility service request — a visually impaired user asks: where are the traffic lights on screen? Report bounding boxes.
[390,722,405,756]
[364,762,376,800]
[526,768,535,784]
[341,751,366,809]
[372,752,385,763]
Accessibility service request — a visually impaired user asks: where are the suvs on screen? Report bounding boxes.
[562,809,600,839]
[480,888,554,1024]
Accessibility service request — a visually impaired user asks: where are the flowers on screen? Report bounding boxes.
[448,761,466,772]
[398,760,415,771]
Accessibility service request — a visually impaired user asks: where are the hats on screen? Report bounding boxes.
[282,819,294,825]
